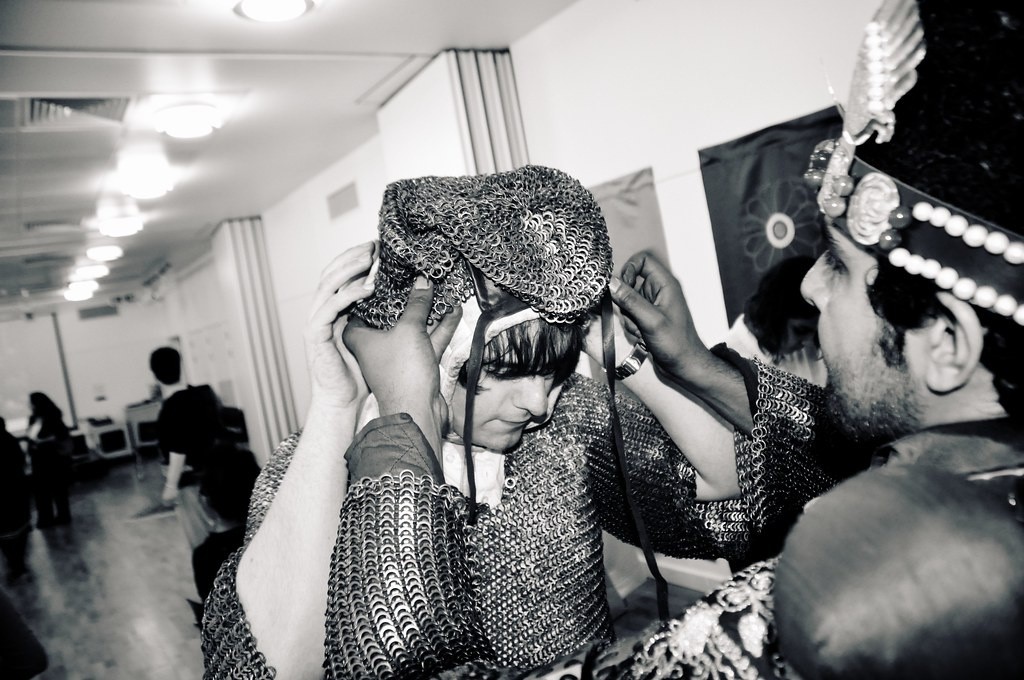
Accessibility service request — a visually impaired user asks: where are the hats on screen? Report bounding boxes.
[800,0,1024,329]
[345,163,670,625]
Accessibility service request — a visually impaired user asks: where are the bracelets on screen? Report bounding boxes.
[615,342,648,382]
[164,484,177,489]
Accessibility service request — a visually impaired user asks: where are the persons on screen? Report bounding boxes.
[148,346,235,634]
[322,1,1024,680]
[726,256,830,388]
[0,392,76,680]
[199,166,749,680]
